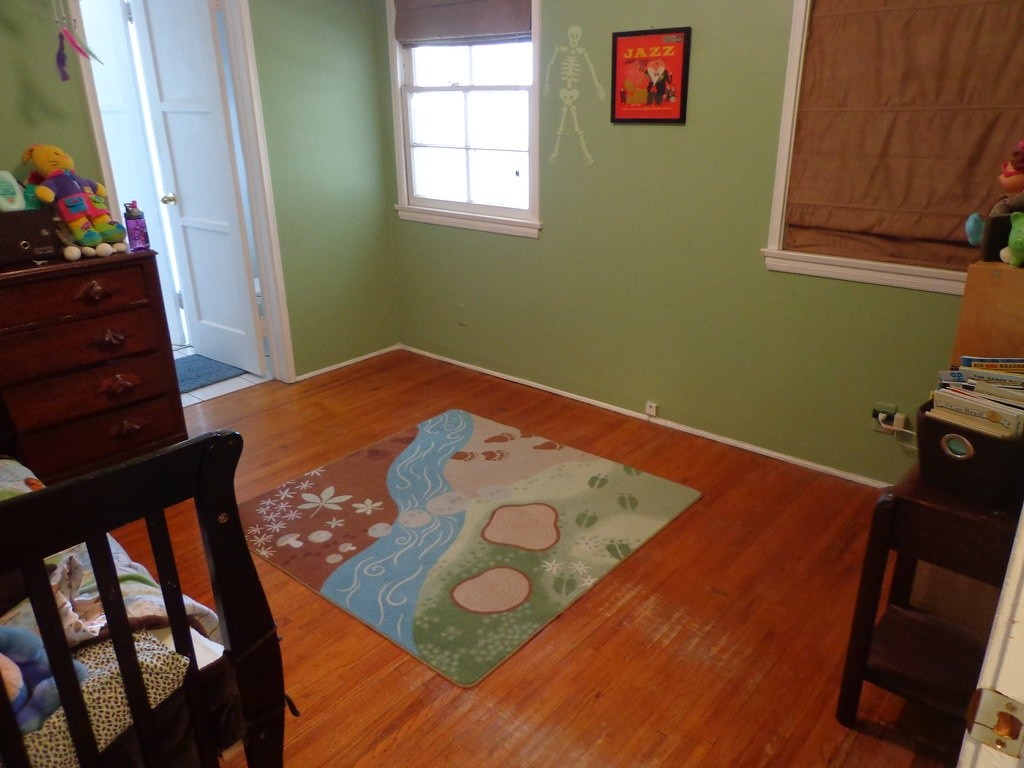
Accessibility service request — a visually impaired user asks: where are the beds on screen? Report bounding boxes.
[0,429,288,768]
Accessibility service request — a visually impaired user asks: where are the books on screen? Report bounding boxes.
[924,356,1024,439]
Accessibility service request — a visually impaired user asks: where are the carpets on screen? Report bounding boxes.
[239,409,704,688]
[175,353,247,394]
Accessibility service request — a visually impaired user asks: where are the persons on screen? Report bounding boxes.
[964,143,1024,262]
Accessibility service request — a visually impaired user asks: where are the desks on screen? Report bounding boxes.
[835,455,1022,726]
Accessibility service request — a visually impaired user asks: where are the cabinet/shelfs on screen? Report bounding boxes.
[950,259,1024,366]
[0,244,193,488]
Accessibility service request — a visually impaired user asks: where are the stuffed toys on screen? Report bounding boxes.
[0,623,88,734]
[20,143,125,246]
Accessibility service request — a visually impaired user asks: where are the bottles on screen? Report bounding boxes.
[123,200,150,252]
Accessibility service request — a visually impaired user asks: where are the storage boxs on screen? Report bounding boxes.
[0,209,61,268]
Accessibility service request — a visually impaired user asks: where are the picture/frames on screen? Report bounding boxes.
[610,27,692,123]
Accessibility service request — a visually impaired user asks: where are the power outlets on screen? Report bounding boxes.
[871,402,896,434]
